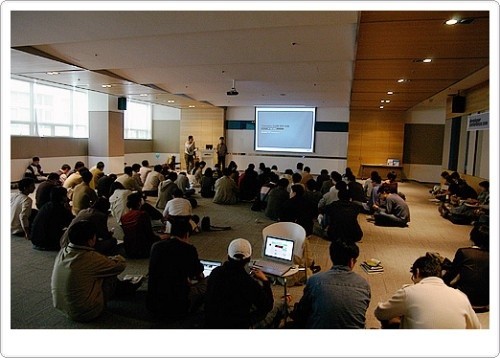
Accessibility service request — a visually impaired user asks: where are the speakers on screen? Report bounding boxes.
[446,94,465,121]
[118,98,127,110]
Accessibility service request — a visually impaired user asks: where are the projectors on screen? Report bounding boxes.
[227,91,238,95]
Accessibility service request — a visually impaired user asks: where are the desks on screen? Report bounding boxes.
[360,163,403,183]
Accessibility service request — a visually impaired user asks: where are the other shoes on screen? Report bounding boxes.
[310,260,321,274]
[281,295,292,303]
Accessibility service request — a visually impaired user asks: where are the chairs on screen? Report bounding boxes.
[258,221,308,329]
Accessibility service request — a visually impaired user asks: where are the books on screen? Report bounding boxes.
[360,259,384,275]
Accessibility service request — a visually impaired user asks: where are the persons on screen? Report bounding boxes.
[374,252,484,329]
[310,168,370,242]
[184,135,198,175]
[193,161,265,205]
[442,224,489,313]
[149,220,274,329]
[155,165,200,236]
[364,171,410,226]
[280,237,374,329]
[50,222,146,324]
[429,171,467,202]
[442,181,489,225]
[78,160,169,261]
[256,162,317,237]
[216,137,230,170]
[10,156,97,252]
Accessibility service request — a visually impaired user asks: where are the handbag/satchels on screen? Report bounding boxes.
[250,198,262,211]
[201,216,210,232]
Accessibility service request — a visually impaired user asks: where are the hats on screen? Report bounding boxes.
[228,238,252,260]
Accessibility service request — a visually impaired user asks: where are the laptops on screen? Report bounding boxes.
[200,259,223,278]
[255,235,294,277]
